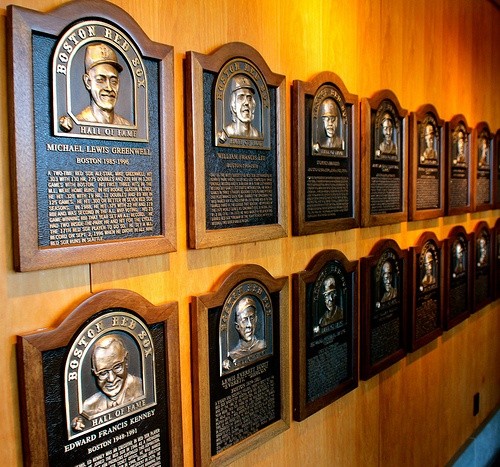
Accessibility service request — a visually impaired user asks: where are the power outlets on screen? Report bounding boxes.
[473,392,479,416]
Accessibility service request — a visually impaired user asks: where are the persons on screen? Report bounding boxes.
[379,113,396,154]
[422,252,436,289]
[477,239,488,268]
[456,132,467,163]
[319,98,343,149]
[82,334,145,415]
[228,297,266,362]
[224,76,260,137]
[478,138,489,166]
[318,277,343,327]
[75,42,133,125]
[380,261,398,304]
[454,243,465,274]
[419,125,438,165]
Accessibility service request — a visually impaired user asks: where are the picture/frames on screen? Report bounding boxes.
[6,0,500,467]
